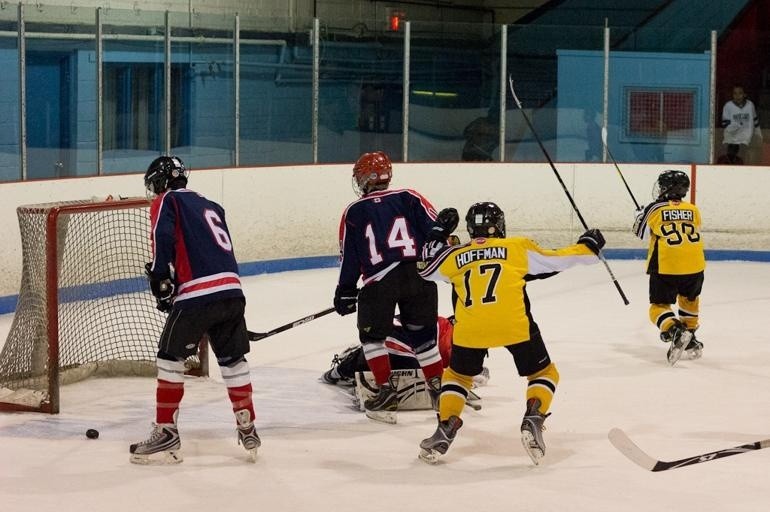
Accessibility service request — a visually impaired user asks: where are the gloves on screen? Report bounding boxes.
[431,208,459,236]
[578,229,605,250]
[334,288,358,316]
[635,206,645,215]
[150,277,178,312]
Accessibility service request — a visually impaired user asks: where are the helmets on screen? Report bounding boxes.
[145,157,187,200]
[351,151,392,198]
[466,202,505,238]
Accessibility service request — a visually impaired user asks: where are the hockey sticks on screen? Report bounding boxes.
[601,126,642,212]
[608,428,770,471]
[509,72,628,304]
[247,307,336,340]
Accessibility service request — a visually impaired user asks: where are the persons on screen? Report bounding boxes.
[323,318,489,391]
[129,157,262,455]
[584,107,603,162]
[721,83,762,165]
[632,170,705,362]
[415,201,605,459]
[461,106,501,161]
[333,152,449,412]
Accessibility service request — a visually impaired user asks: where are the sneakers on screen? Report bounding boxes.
[322,370,338,384]
[686,329,703,350]
[427,377,440,411]
[364,385,397,411]
[661,323,687,360]
[521,398,551,454]
[420,414,463,454]
[235,424,261,450]
[652,171,689,200]
[130,422,181,454]
